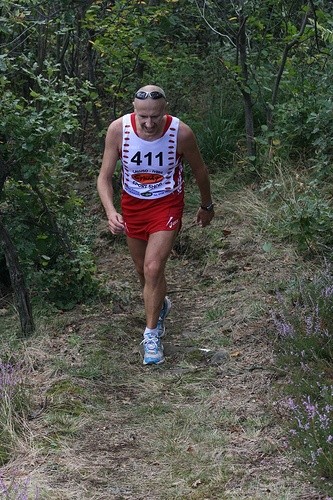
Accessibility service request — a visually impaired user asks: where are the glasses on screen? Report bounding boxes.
[134,91,167,102]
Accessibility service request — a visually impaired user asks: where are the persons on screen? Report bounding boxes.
[97,85,215,364]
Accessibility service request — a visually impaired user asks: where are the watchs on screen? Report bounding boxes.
[201,203,214,211]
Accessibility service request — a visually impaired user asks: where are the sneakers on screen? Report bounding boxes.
[156,296,172,338]
[143,332,166,365]
